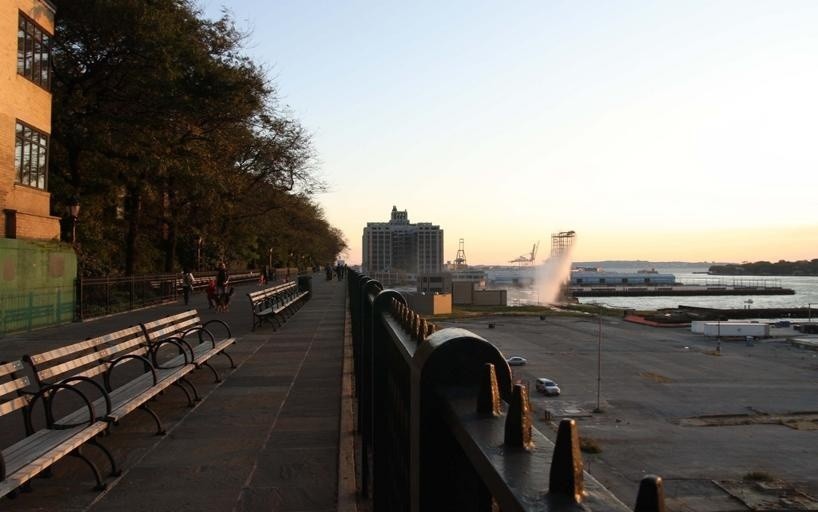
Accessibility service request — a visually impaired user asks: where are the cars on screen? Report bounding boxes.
[507,357,528,366]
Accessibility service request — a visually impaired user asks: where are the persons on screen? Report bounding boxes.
[178,265,196,304]
[324,263,344,281]
[216,262,230,293]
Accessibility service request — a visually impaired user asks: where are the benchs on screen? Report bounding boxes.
[22,324,196,478]
[244,279,310,335]
[139,309,237,402]
[0,360,109,512]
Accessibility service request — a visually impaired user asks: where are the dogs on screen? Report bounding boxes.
[210,287,234,314]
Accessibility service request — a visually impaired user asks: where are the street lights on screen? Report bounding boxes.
[69,199,81,242]
[268,250,305,279]
[197,236,203,274]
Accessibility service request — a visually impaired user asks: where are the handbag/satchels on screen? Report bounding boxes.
[185,275,192,285]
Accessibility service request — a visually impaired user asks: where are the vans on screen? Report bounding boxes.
[536,378,560,396]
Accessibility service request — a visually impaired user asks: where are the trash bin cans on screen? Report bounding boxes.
[297,276,313,299]
